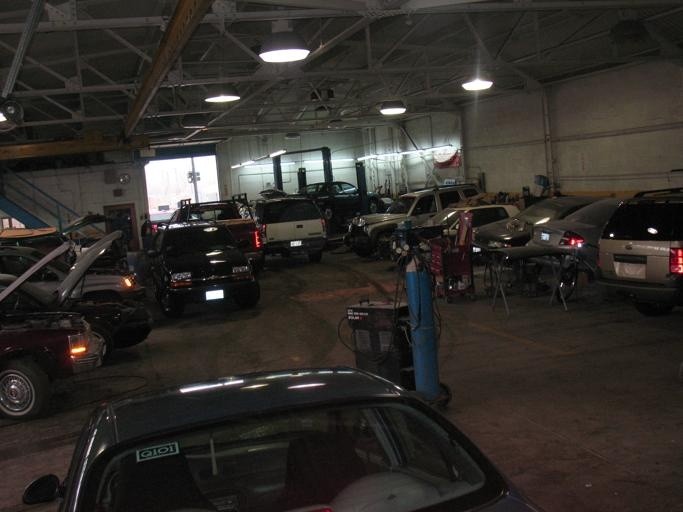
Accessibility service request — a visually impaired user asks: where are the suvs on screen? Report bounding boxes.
[342,185,483,256]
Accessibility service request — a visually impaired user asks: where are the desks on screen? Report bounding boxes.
[484,243,574,316]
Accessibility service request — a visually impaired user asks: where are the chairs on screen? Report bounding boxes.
[120,450,218,511]
[286,434,379,509]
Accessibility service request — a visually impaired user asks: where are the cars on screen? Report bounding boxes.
[23,365,542,511]
[150,223,260,317]
[1,210,152,420]
[411,187,682,315]
[141,190,326,261]
[301,181,385,221]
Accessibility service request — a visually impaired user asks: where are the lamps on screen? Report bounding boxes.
[284,132,302,139]
[257,30,311,64]
[380,100,408,115]
[462,73,494,90]
[310,90,322,102]
[181,114,206,129]
[325,88,335,99]
[269,148,286,158]
[203,83,241,104]
[230,160,254,170]
[327,120,347,130]
